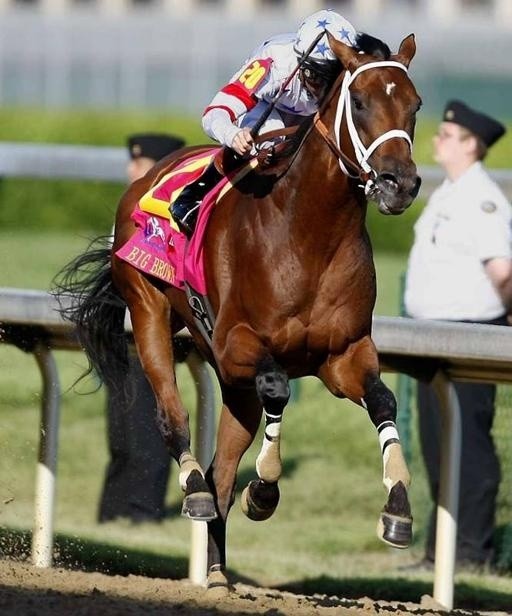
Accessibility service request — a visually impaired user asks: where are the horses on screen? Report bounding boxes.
[48,28,423,599]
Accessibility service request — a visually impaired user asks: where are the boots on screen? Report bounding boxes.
[168,144,241,235]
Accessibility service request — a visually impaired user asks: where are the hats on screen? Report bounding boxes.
[127,135,186,163]
[442,99,505,148]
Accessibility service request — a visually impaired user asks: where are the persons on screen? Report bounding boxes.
[101,134,186,523]
[398,100,511,572]
[168,11,356,236]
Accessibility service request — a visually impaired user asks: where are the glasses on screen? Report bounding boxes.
[297,57,336,89]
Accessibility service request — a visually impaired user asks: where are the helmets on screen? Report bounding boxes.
[293,9,359,69]
